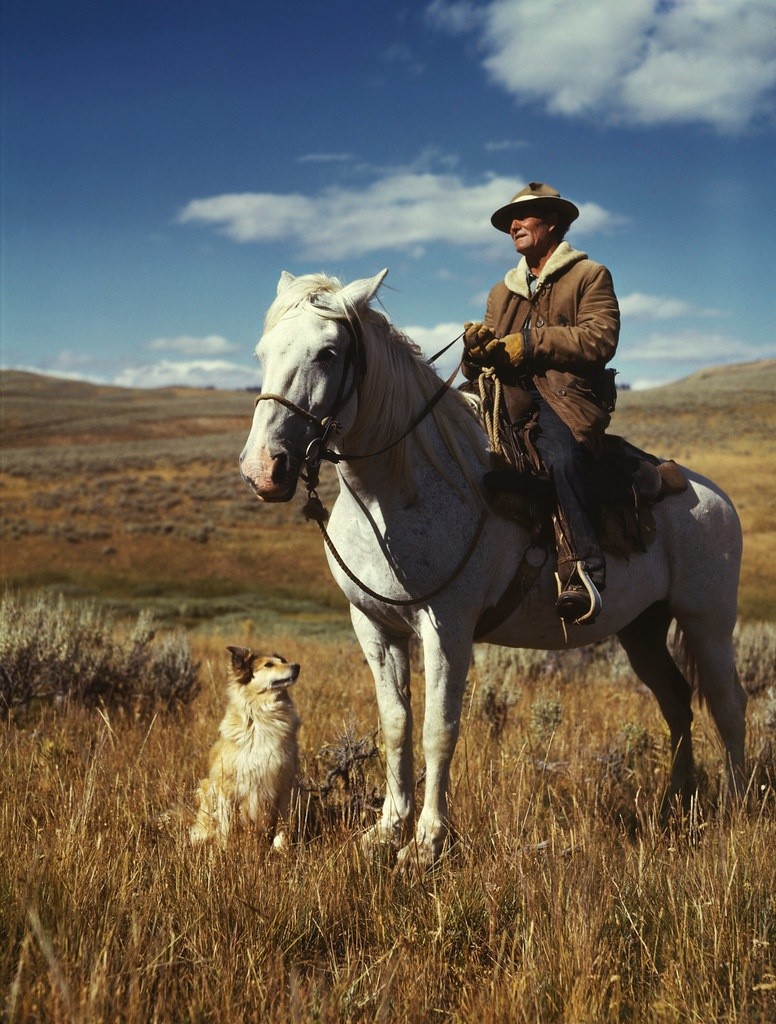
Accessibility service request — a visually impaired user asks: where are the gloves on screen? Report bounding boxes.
[463,322,524,372]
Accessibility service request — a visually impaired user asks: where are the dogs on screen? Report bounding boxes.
[189,645,302,852]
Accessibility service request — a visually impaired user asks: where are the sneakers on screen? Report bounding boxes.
[556,583,596,627]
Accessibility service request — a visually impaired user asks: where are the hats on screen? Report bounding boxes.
[491,182,579,234]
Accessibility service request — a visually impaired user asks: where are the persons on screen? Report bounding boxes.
[456,183,621,623]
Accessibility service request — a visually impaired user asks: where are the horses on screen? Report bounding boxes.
[235,265,750,867]
